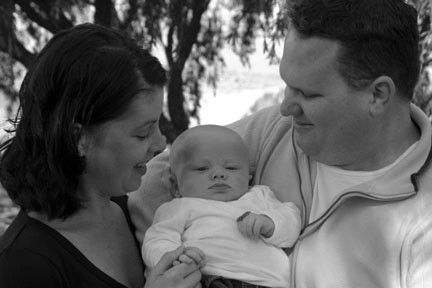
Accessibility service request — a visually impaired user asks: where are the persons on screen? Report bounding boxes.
[128,0,432,288]
[140,122,302,288]
[0,23,202,288]
